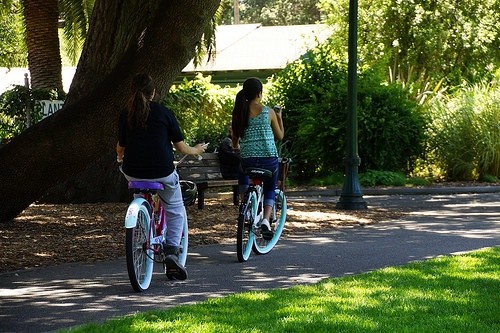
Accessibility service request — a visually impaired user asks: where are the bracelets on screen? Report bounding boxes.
[117,155,123,162]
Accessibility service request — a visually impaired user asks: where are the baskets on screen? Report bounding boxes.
[179,180,197,206]
[278,158,290,181]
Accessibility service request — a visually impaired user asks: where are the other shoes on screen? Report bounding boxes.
[163,244,187,280]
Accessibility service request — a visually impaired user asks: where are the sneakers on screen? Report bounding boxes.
[245,210,251,220]
[261,219,272,240]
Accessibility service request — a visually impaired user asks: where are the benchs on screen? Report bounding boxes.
[174,153,241,209]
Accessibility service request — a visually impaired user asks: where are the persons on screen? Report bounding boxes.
[116,73,208,280]
[230,77,284,240]
[218,126,250,207]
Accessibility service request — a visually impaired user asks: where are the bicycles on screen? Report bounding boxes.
[235,152,293,263]
[116,151,203,293]
